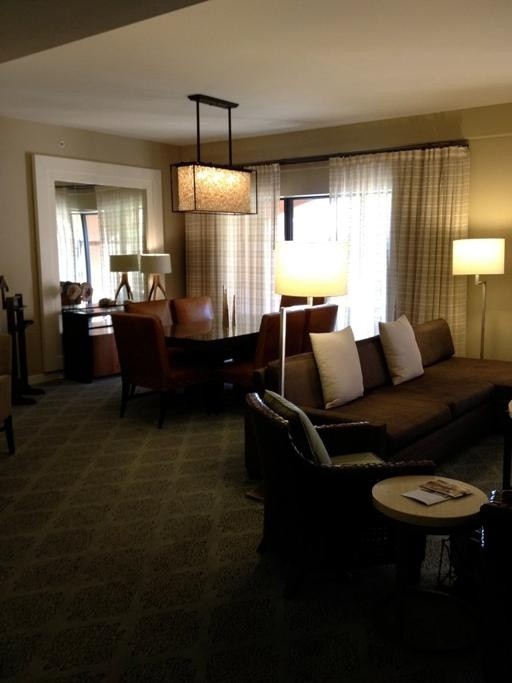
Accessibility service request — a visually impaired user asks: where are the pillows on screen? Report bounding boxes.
[265,389,332,465]
[379,313,425,389]
[306,330,365,411]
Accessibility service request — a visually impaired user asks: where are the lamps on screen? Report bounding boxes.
[274,240,347,306]
[170,94,258,215]
[142,253,171,302]
[110,255,140,300]
[452,238,506,362]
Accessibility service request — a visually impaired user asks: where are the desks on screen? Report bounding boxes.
[371,475,491,619]
[62,305,127,383]
[155,314,260,413]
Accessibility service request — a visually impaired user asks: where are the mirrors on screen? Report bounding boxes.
[54,185,147,311]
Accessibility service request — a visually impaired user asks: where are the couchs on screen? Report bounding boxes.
[264,318,512,466]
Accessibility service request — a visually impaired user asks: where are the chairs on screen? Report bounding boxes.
[278,292,328,313]
[218,310,306,403]
[301,305,341,352]
[124,300,182,397]
[110,313,206,429]
[0,332,17,456]
[249,393,434,589]
[166,295,218,323]
